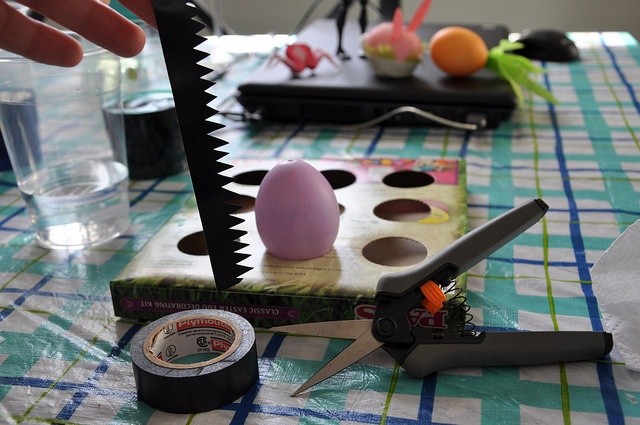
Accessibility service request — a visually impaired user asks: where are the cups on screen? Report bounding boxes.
[90,19,184,181]
[1,33,132,252]
[3,56,44,173]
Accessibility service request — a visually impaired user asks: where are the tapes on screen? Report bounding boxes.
[130,309,260,414]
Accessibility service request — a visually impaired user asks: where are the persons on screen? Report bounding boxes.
[0,0,157,66]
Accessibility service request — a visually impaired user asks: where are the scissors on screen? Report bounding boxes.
[267,197,614,398]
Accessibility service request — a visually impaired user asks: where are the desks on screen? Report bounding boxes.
[1,32,639,424]
